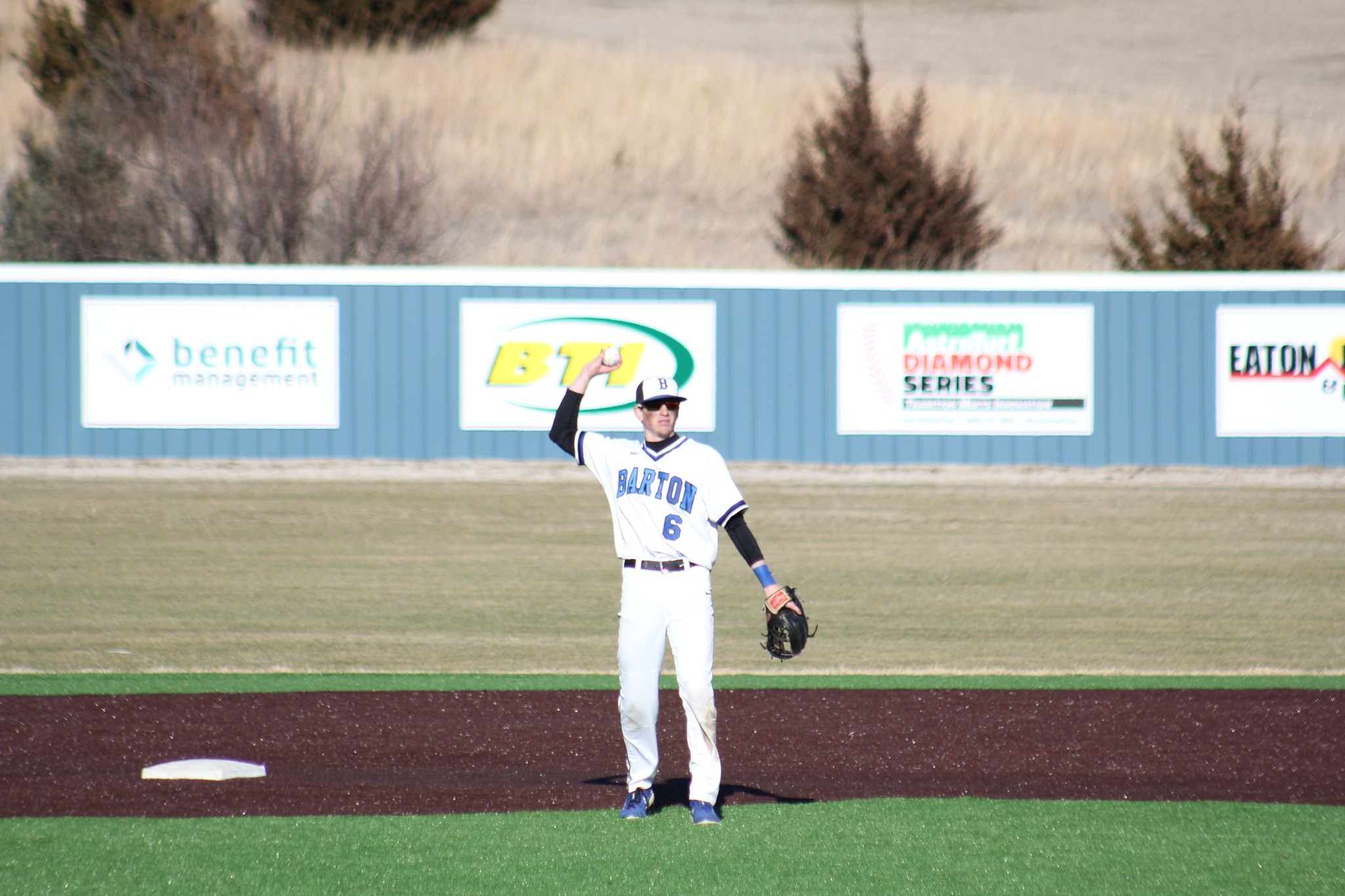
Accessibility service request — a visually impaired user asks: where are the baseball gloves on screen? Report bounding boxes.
[759,585,819,664]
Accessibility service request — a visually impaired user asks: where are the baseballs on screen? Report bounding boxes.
[601,345,623,367]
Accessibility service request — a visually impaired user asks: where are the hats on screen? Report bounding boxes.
[636,376,687,404]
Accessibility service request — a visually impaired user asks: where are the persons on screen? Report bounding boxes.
[548,349,802,822]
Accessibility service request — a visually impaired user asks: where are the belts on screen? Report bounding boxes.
[624,559,698,574]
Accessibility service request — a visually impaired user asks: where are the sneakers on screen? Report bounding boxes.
[689,800,720,825]
[619,787,654,818]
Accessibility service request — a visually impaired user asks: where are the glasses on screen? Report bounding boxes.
[637,402,680,412]
